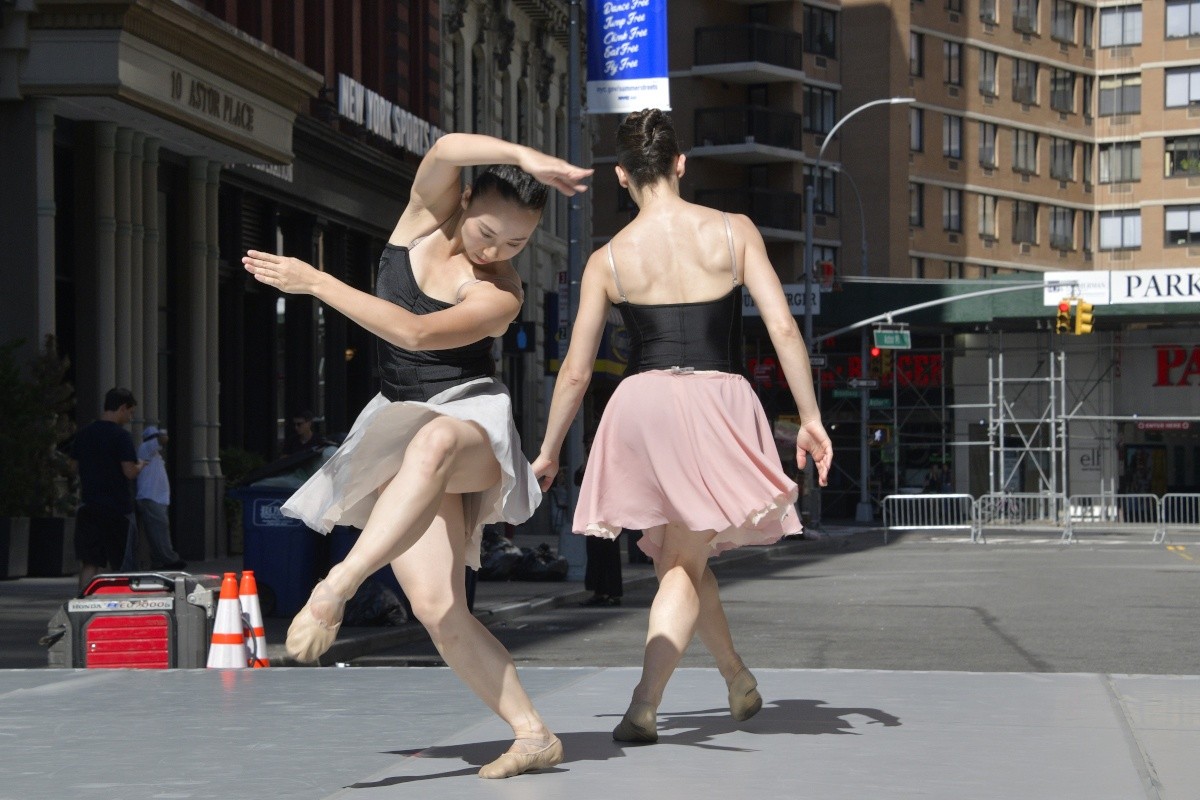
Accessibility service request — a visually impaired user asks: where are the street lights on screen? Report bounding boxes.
[828,165,875,525]
[796,96,916,527]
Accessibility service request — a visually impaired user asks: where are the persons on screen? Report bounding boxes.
[54,387,188,597]
[241,132,594,779]
[528,108,832,743]
[923,462,955,493]
[279,408,324,461]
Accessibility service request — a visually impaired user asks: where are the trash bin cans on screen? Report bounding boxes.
[326,523,410,622]
[39,570,222,671]
[229,483,325,625]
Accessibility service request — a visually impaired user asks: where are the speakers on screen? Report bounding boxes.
[49,572,220,669]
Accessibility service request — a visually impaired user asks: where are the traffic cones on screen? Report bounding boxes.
[204,573,253,668]
[236,570,273,667]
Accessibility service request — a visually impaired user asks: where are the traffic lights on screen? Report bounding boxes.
[871,426,890,446]
[870,346,881,375]
[1057,301,1075,336]
[1076,302,1095,338]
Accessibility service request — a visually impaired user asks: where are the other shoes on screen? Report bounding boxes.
[162,560,188,570]
[579,592,621,607]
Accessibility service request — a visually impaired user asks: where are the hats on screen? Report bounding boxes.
[142,426,160,441]
